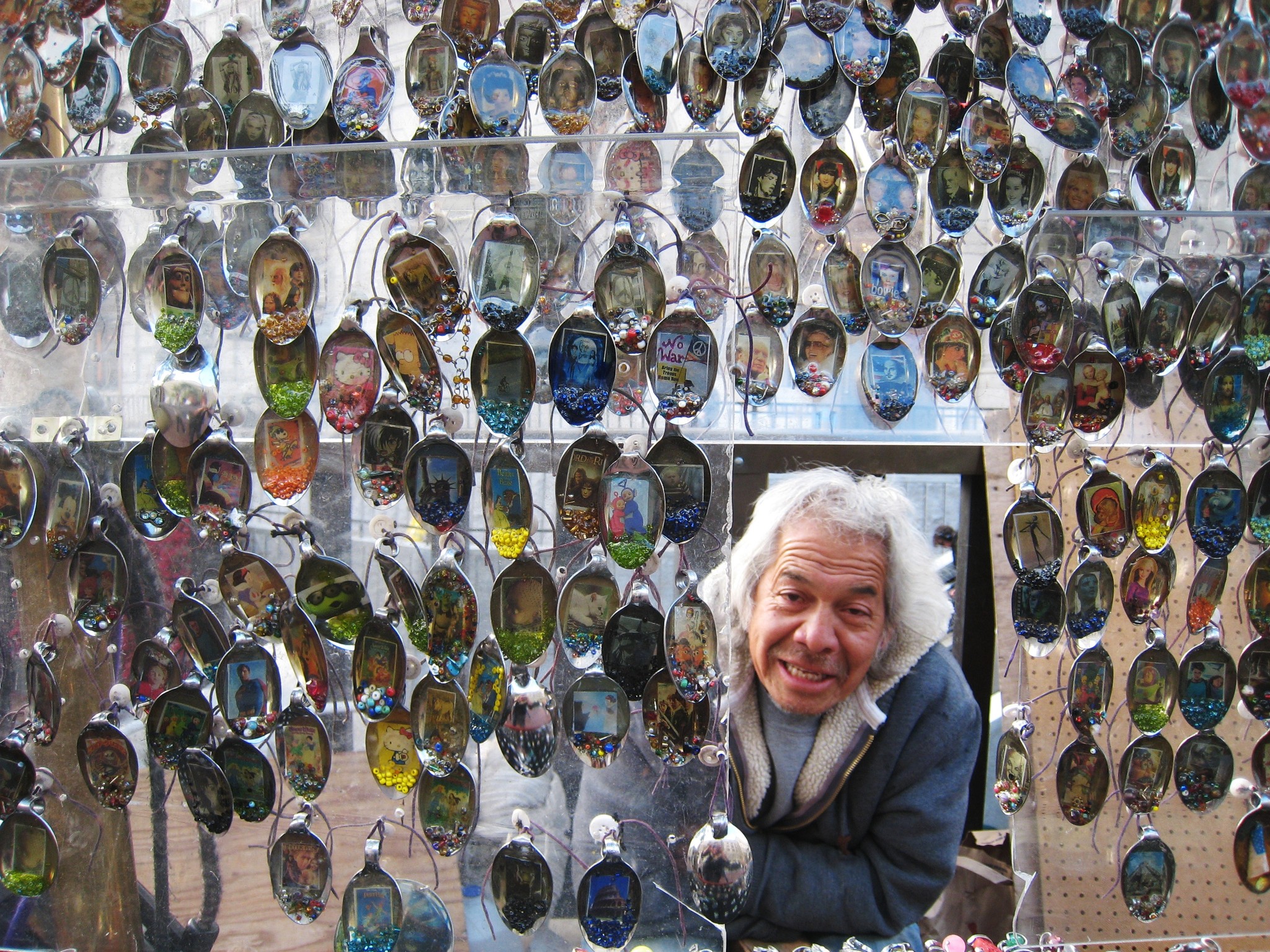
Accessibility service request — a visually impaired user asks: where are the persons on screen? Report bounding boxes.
[1064,75,1095,110]
[1064,173,1096,216]
[994,171,1027,217]
[737,340,769,381]
[940,168,970,209]
[803,330,834,372]
[569,465,981,952]
[263,292,281,316]
[796,2,1270,918]
[879,358,906,388]
[906,102,938,149]
[138,662,166,702]
[235,665,268,718]
[0,0,790,952]
[298,859,319,887]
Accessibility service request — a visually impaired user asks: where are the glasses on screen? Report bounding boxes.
[803,341,829,350]
[149,168,169,176]
[1170,77,1175,87]
